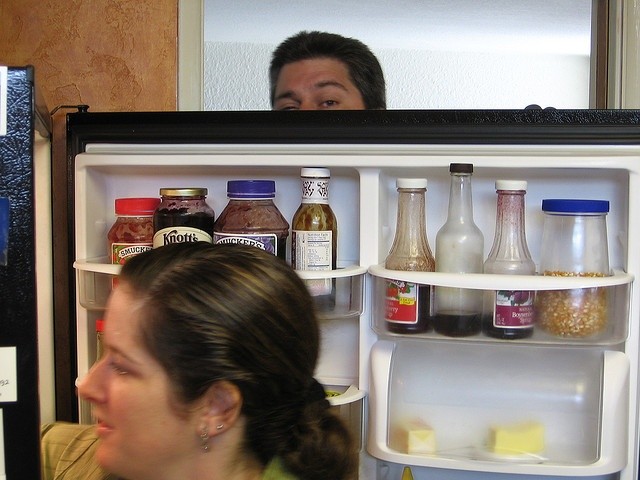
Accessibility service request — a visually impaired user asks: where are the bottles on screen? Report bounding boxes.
[482,179,537,337]
[535,198,613,338]
[212,180,289,264]
[292,167,338,312]
[108,198,162,289]
[384,179,435,333]
[433,164,485,336]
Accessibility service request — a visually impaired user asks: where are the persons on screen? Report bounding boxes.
[265,26,388,113]
[39,240,354,480]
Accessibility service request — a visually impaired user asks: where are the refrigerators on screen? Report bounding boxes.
[0,64,640,480]
